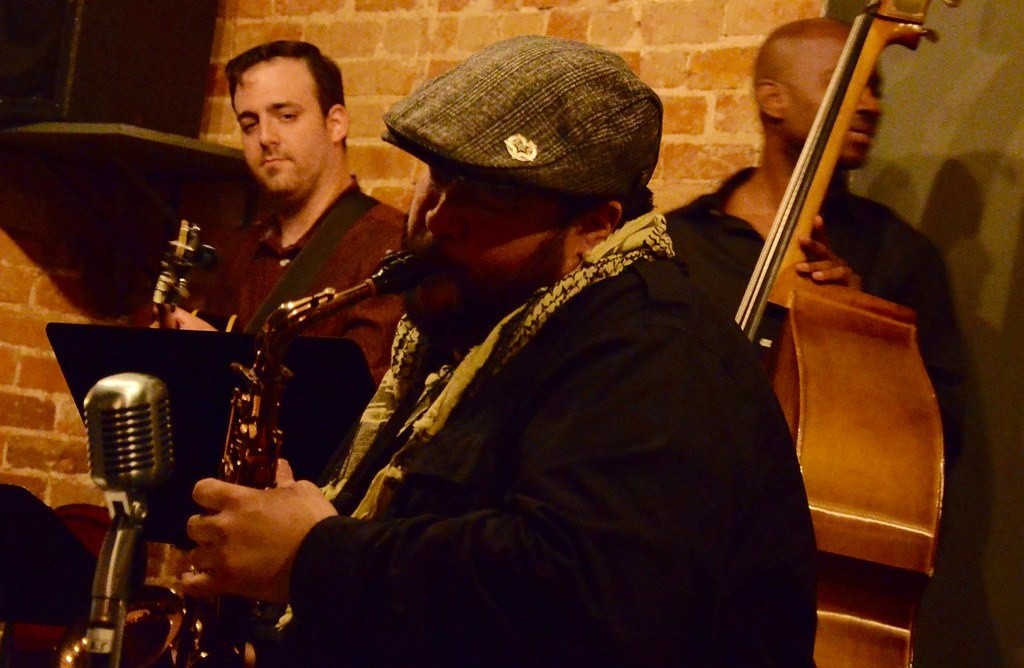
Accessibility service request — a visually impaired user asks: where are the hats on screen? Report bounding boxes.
[382,34,666,197]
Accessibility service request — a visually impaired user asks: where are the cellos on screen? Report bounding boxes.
[735,0,959,668]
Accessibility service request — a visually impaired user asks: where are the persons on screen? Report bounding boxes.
[180,32,815,667]
[145,41,411,392]
[652,15,970,526]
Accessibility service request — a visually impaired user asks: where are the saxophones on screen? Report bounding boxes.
[56,247,434,668]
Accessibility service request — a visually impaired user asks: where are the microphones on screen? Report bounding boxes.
[84,371,173,486]
